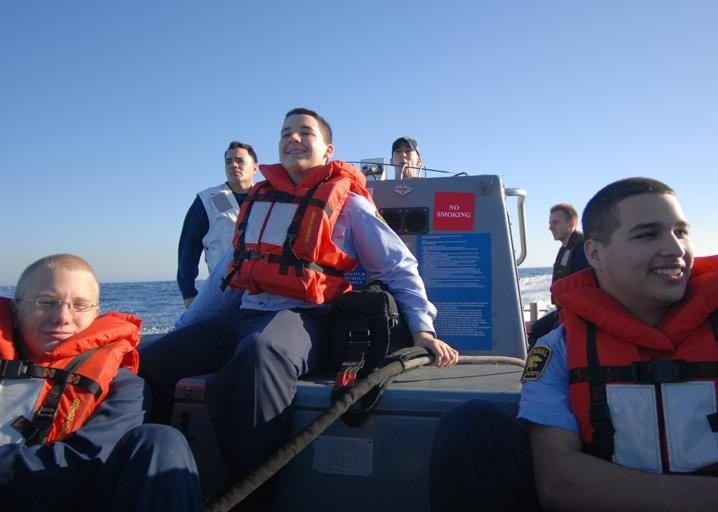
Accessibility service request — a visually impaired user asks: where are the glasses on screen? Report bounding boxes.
[16,295,101,311]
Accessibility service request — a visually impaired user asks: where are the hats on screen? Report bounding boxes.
[392,137,421,158]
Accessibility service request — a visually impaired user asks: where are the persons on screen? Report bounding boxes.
[390,135,423,180]
[135,108,459,510]
[0,253,205,512]
[514,174,718,512]
[526,203,590,355]
[176,141,260,311]
